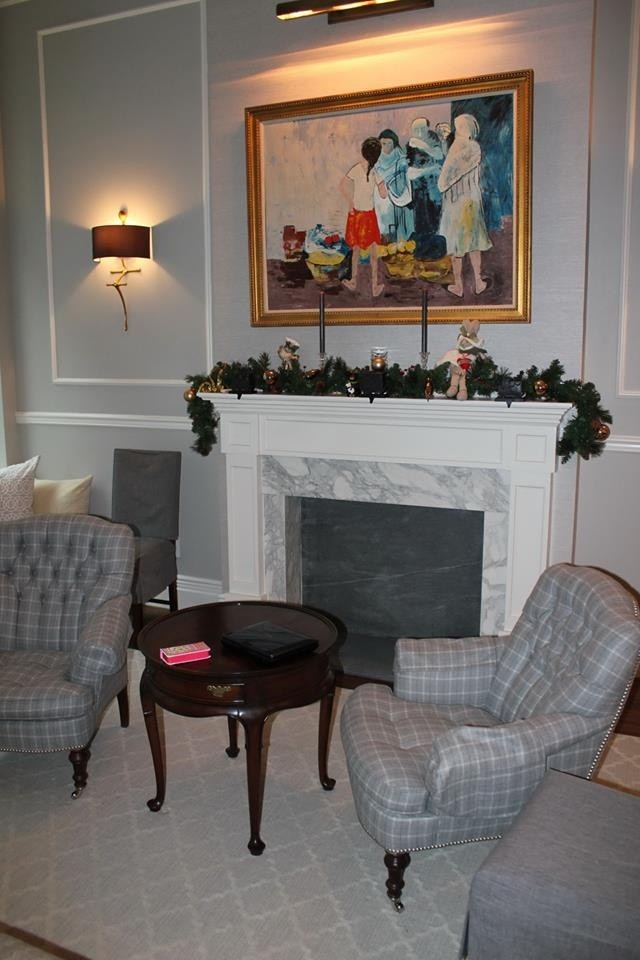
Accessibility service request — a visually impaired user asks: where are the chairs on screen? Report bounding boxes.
[0,512,136,797]
[109,449,182,612]
[340,563,640,913]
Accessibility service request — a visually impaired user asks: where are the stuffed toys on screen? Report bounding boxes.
[434,320,481,401]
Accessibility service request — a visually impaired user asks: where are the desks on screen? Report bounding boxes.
[136,601,338,856]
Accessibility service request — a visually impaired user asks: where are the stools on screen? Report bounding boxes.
[456,765,640,960]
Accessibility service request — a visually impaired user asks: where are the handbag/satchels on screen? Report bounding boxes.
[222,619,320,663]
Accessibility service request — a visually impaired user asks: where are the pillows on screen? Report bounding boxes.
[33,475,93,515]
[0,455,40,521]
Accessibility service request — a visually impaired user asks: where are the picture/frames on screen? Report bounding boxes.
[245,68,534,328]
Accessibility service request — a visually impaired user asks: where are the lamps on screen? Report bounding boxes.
[276,0,434,25]
[92,211,151,331]
